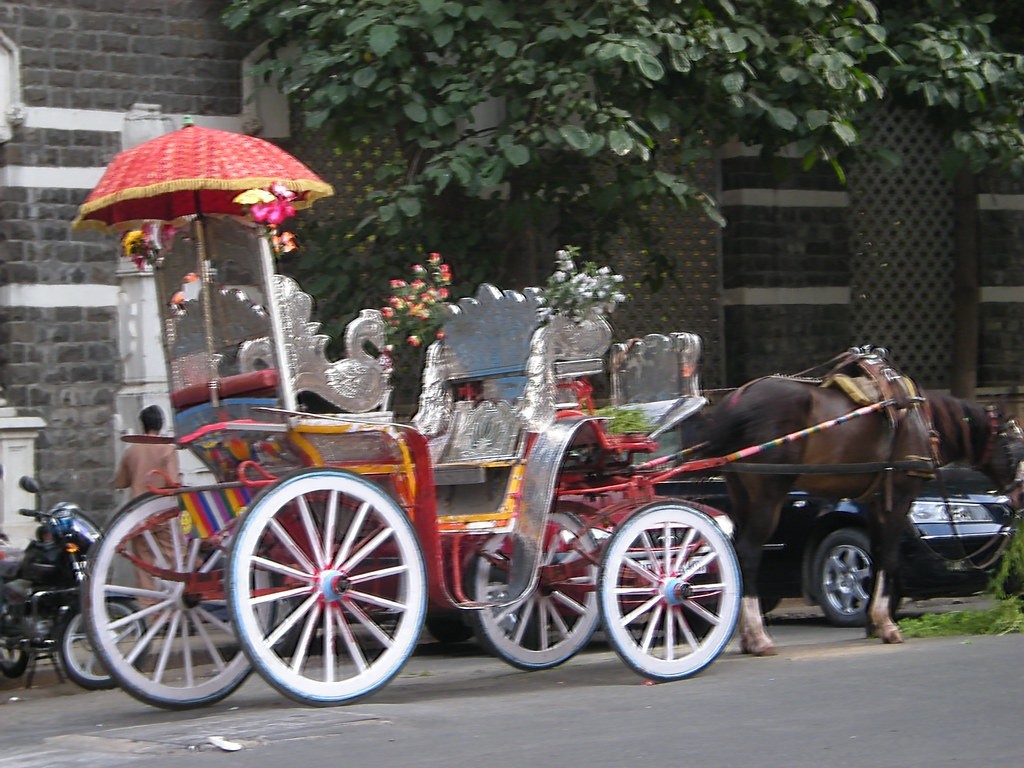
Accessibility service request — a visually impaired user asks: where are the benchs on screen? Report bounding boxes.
[410,283,614,468]
[610,333,708,441]
[155,216,393,426]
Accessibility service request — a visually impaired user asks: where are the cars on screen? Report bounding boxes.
[644,462,1017,627]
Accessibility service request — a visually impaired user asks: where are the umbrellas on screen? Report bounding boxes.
[69,114,333,405]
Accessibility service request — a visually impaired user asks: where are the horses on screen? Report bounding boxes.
[700,373,1024,656]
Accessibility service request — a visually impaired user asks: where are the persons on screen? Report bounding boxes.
[111,404,197,636]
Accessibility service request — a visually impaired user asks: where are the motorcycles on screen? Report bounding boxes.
[1,476,152,688]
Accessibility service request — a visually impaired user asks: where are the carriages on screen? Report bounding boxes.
[71,110,1024,711]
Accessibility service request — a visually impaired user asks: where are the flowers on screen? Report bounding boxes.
[542,245,627,323]
[378,253,450,384]
[122,222,179,271]
[170,271,197,313]
[249,180,298,261]
